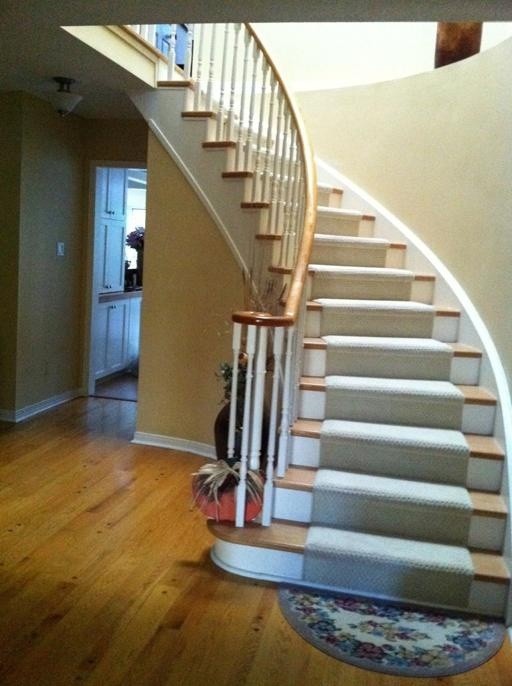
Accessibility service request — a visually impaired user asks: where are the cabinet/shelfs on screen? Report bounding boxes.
[95,168,128,295]
[94,297,143,379]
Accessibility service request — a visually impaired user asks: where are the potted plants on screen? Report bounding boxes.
[214,359,269,470]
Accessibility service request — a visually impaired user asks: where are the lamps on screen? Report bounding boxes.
[48,76,84,118]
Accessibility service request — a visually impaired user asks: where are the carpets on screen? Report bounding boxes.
[276,581,506,677]
[202,89,474,608]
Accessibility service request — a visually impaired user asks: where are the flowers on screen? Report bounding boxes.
[125,226,145,252]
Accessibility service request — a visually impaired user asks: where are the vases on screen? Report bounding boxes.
[137,252,143,286]
